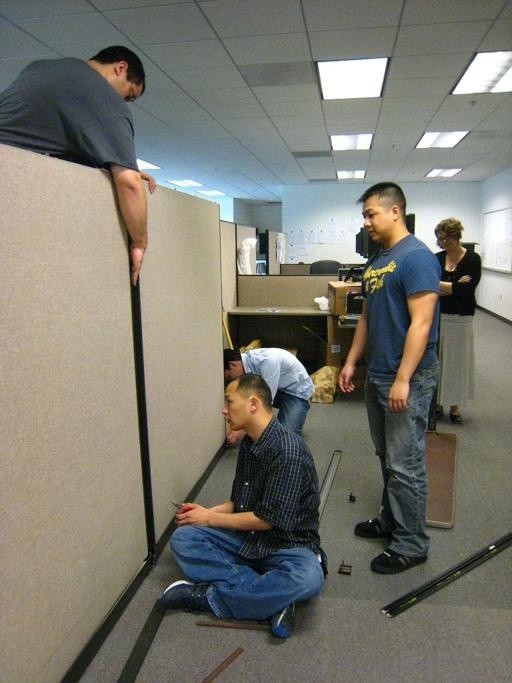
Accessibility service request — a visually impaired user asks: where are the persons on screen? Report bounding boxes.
[2,45,155,284]
[430,214,480,423]
[158,373,329,639]
[337,181,443,575]
[222,346,312,444]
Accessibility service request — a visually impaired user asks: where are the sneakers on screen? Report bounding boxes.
[370,549,427,574]
[270,600,296,638]
[354,518,385,539]
[160,580,210,614]
[450,411,462,424]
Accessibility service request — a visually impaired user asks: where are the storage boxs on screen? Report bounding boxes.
[327,279,363,315]
